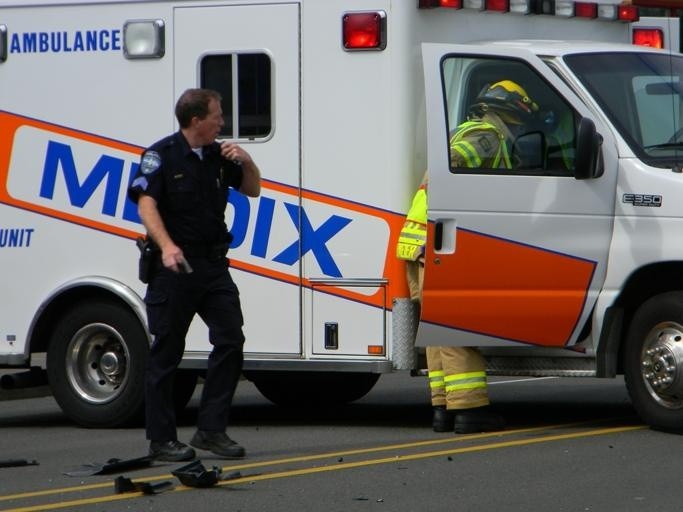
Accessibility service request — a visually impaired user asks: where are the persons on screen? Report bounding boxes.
[395,80,538,434]
[126,87,261,462]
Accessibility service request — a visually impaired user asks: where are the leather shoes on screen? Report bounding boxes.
[149,429,246,462]
[433,408,513,433]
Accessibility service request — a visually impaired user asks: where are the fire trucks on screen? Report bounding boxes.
[0,2,683,432]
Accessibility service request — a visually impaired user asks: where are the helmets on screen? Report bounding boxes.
[469,78,539,121]
